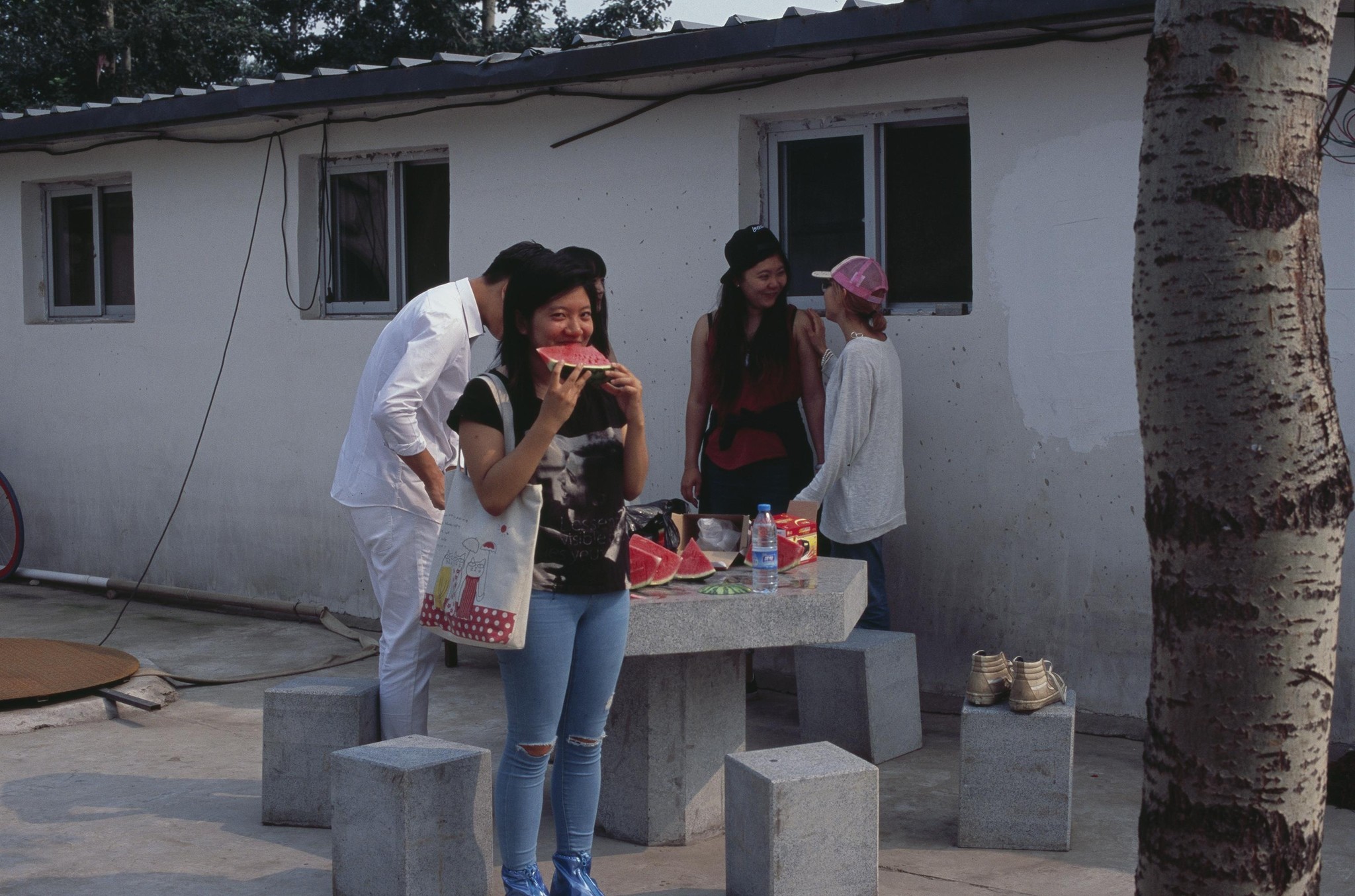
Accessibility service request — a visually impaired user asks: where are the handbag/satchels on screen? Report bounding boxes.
[420,373,544,650]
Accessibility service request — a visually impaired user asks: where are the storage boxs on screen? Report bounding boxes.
[670,500,819,570]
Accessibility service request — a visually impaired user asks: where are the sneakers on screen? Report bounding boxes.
[1009,656,1067,711]
[966,650,1014,705]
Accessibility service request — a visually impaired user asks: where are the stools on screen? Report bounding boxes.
[794,628,923,764]
[725,741,879,896]
[261,676,380,827]
[957,690,1076,851]
[329,734,493,896]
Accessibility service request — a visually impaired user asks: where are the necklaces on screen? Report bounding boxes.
[745,351,749,367]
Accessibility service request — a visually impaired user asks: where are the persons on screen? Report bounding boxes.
[446,254,650,896]
[792,254,908,632]
[557,246,620,365]
[329,239,556,743]
[679,221,826,700]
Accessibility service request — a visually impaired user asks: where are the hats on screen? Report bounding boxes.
[811,256,889,304]
[721,224,783,284]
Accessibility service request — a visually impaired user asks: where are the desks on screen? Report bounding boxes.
[594,555,867,846]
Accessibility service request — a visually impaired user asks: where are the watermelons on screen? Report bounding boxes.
[536,342,615,385]
[629,533,716,590]
[743,534,804,573]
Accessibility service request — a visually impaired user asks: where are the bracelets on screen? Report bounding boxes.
[815,462,825,470]
[820,347,834,368]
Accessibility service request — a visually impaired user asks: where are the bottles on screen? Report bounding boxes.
[752,504,779,593]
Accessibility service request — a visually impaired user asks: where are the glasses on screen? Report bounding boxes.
[821,281,832,292]
